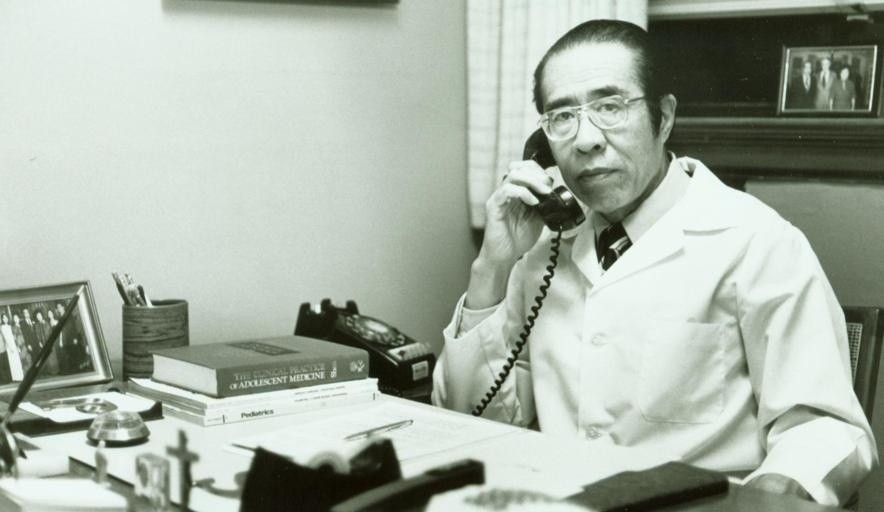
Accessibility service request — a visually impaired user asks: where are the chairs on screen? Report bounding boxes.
[837,302,884,429]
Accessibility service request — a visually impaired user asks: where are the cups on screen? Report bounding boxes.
[121,300,189,382]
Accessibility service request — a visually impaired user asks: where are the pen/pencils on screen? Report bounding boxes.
[4,282,87,430]
[112,271,153,307]
[345,420,413,441]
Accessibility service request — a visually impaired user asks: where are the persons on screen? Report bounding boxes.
[430,19,881,510]
[0,302,82,383]
[813,57,838,110]
[828,65,857,111]
[790,60,818,109]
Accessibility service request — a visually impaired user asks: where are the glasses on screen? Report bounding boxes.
[536,97,646,140]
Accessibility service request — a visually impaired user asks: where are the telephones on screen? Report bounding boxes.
[523,128,585,232]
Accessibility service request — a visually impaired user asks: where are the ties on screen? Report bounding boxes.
[600,224,630,272]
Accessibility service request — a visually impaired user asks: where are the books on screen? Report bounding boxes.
[124,334,382,428]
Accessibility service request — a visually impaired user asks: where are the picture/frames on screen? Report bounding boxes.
[0,282,113,394]
[775,44,878,116]
